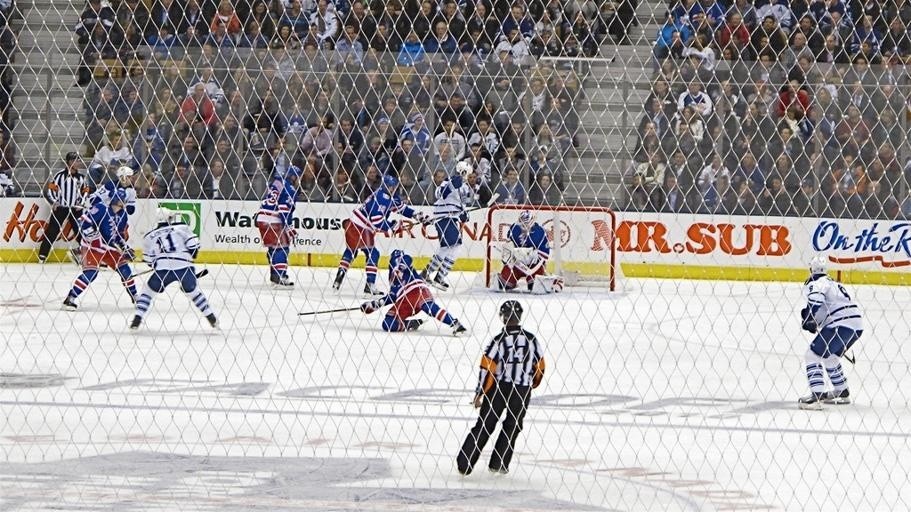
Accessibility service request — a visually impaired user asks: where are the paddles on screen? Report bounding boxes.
[297,305,360,316]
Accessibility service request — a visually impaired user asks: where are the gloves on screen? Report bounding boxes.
[451,173,463,189]
[413,210,425,222]
[361,300,376,315]
[122,244,136,262]
[802,317,817,334]
[284,226,297,243]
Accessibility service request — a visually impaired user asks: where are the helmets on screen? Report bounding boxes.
[284,164,302,179]
[390,249,405,257]
[456,161,474,182]
[382,176,398,187]
[116,166,133,177]
[66,152,81,161]
[518,210,535,230]
[499,300,523,318]
[109,189,127,203]
[809,257,828,274]
[155,207,176,223]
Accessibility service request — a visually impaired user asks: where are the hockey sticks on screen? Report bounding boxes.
[489,241,544,274]
[816,326,857,365]
[134,257,209,279]
[398,193,502,227]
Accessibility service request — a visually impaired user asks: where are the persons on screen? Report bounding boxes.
[363,248,467,335]
[75,0,637,210]
[797,256,863,410]
[633,0,911,221]
[60,188,140,309]
[453,300,545,477]
[497,210,552,291]
[330,176,430,296]
[127,207,216,329]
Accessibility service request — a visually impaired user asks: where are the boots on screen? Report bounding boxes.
[62,289,78,308]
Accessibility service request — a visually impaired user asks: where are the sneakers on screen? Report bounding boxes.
[207,313,221,331]
[798,393,827,405]
[363,283,384,295]
[130,316,142,330]
[828,389,849,399]
[331,281,341,289]
[412,270,467,338]
[69,247,80,264]
[277,274,293,285]
